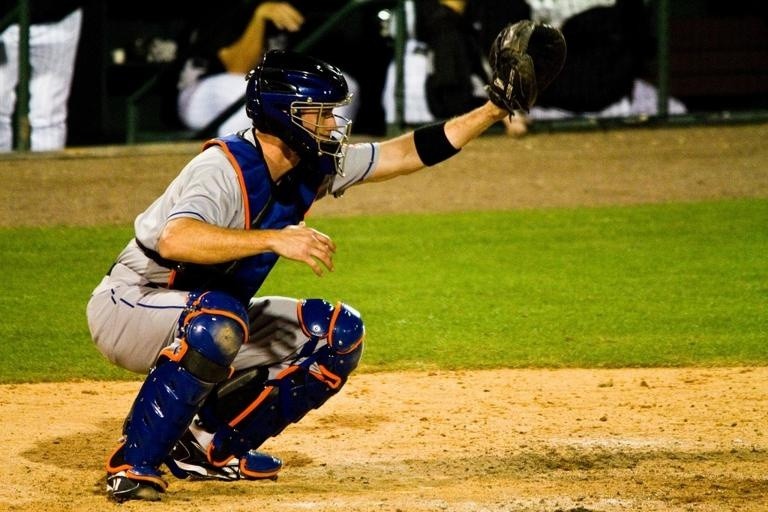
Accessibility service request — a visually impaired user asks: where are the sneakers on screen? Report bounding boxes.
[172,426,278,481]
[105,469,161,504]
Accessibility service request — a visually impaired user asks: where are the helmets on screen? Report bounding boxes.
[246,50,355,178]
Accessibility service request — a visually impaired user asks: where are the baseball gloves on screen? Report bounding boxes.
[486,21,568,122]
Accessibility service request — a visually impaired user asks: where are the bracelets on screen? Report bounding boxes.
[415,120,462,166]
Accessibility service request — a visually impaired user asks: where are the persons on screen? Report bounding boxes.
[0,0,86,151]
[86,17,566,502]
[176,2,689,141]
